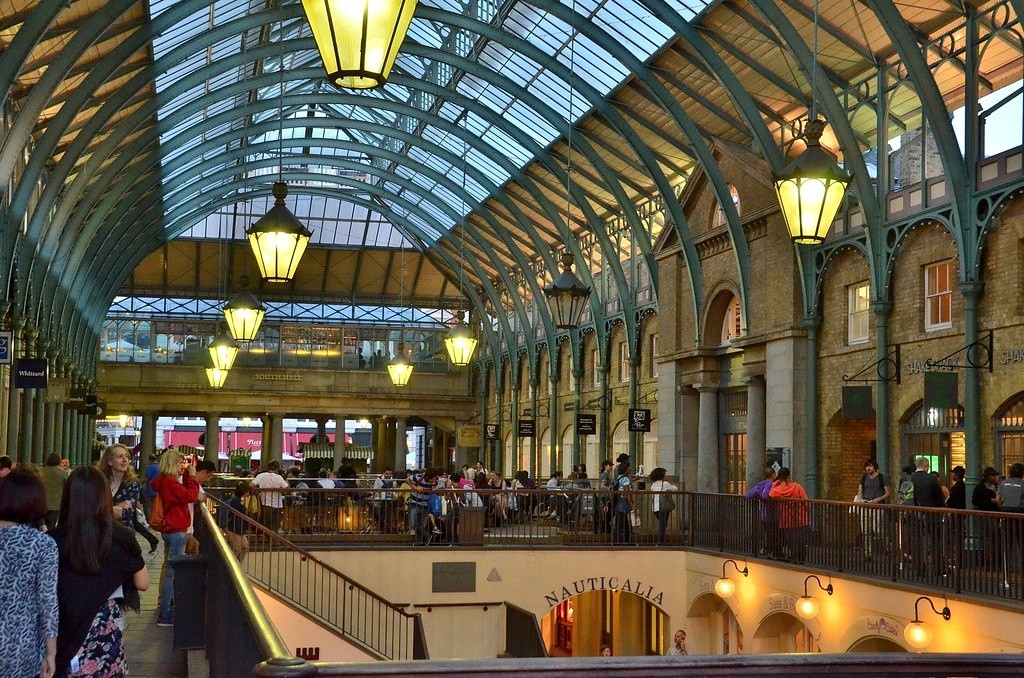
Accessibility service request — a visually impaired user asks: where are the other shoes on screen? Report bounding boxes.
[432,527,442,534]
[148,540,159,553]
[156,617,173,627]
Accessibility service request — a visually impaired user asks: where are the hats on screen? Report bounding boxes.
[984,467,1001,476]
[601,460,614,465]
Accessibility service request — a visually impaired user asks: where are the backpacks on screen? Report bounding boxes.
[148,492,164,531]
[896,480,915,517]
[608,476,631,501]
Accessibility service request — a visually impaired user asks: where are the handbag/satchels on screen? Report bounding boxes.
[659,480,676,513]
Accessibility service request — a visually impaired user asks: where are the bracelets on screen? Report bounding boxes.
[681,649,686,651]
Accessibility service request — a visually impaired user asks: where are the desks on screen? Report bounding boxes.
[361,496,400,535]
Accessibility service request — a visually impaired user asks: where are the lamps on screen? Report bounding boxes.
[539,0,592,330]
[770,0,856,248]
[244,0,315,286]
[714,560,749,597]
[387,186,415,387]
[903,597,951,649]
[204,201,267,389]
[301,0,418,90]
[443,109,481,367]
[795,575,833,619]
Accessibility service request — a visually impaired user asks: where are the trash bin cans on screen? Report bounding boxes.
[448,505,487,546]
[165,555,208,650]
[628,477,679,535]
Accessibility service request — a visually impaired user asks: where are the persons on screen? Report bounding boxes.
[650,468,678,547]
[372,461,508,535]
[141,446,216,627]
[0,444,159,678]
[546,464,590,528]
[899,459,966,577]
[600,645,610,656]
[666,630,689,656]
[226,482,250,562]
[359,346,383,369]
[769,467,811,563]
[858,459,891,560]
[972,463,1024,573]
[225,458,371,532]
[599,453,639,544]
[746,467,775,554]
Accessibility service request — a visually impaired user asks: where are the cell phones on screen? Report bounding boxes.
[192,455,195,467]
